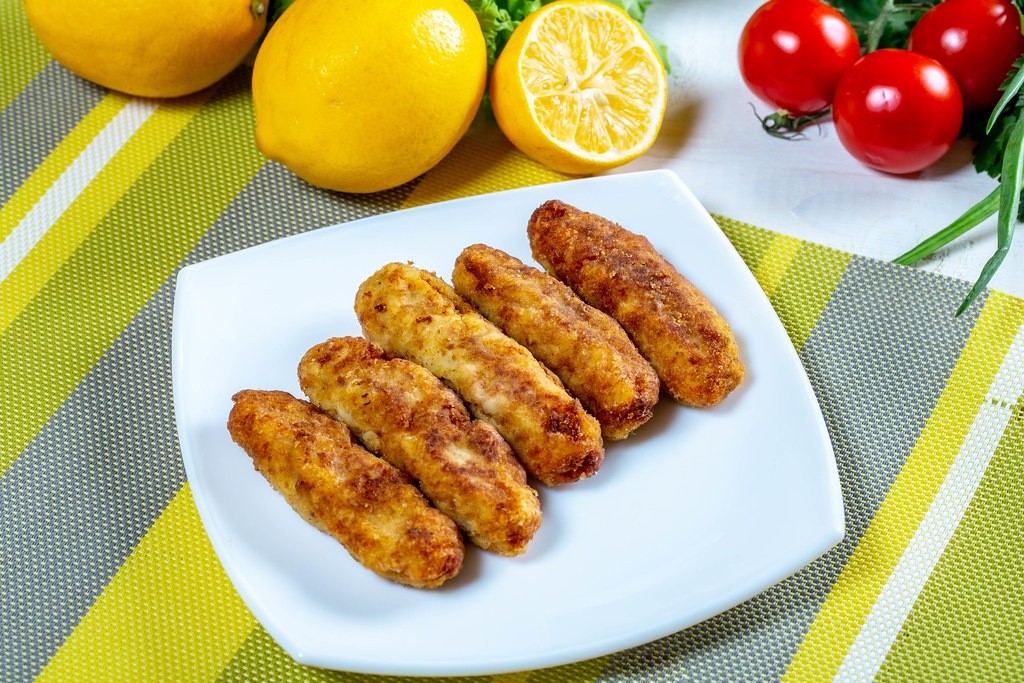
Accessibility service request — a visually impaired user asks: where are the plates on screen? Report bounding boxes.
[172,169,847,677]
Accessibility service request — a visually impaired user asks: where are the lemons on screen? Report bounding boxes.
[21,2,669,195]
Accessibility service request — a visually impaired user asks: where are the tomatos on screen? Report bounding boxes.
[741,0,1023,173]
